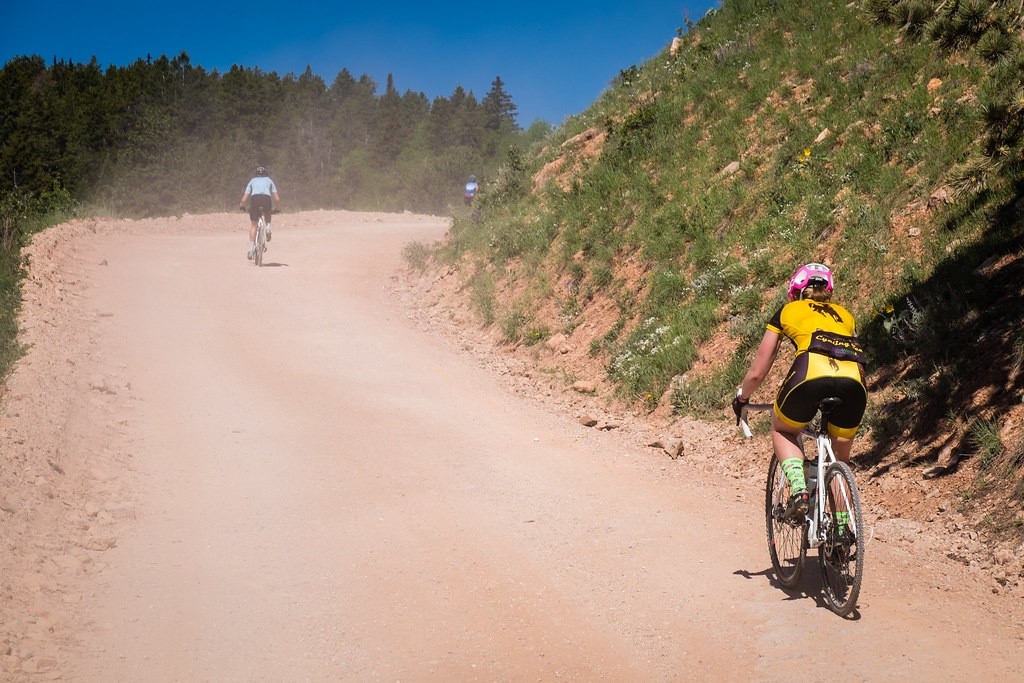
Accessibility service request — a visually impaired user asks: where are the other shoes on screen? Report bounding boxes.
[265,229,272,242]
[248,248,254,260]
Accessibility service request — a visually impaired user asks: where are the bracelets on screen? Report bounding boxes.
[737,388,748,401]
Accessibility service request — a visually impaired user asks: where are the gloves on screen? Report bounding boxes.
[275,209,281,213]
[240,207,245,211]
[731,396,748,418]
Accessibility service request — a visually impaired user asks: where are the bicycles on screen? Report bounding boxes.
[737,396,873,617]
[243,205,281,265]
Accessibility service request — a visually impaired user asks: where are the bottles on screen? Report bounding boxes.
[808,455,818,504]
[803,456,810,485]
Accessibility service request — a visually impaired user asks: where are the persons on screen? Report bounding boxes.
[464,175,479,207]
[240,166,280,260]
[732,263,868,569]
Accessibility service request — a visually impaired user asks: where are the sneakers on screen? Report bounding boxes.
[783,488,810,522]
[829,535,852,568]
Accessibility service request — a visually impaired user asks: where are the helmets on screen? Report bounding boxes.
[469,175,477,182]
[787,263,834,302]
[256,167,268,176]
